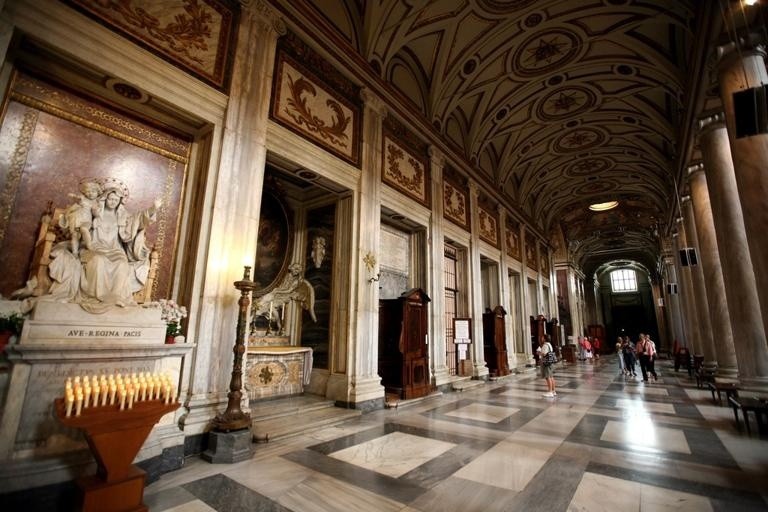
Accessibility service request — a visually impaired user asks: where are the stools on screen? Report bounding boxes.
[689,365,765,442]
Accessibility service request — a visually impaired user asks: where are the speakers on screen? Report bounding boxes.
[689,249,697,265]
[679,250,688,266]
[731,87,758,140]
[668,285,671,294]
[673,285,677,294]
[756,84,768,134]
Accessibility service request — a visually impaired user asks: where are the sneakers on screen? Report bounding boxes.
[620,371,658,382]
[540,391,556,398]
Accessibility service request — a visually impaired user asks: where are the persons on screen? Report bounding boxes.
[615,336,625,375]
[581,336,594,362]
[644,334,657,378]
[59,174,104,260]
[592,338,600,360]
[620,335,637,376]
[250,261,304,323]
[635,333,658,382]
[535,334,557,398]
[58,179,164,309]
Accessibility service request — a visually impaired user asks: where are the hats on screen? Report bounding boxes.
[638,333,650,339]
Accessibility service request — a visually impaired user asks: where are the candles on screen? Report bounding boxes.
[62,371,177,421]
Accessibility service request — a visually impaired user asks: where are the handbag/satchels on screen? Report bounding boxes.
[541,343,558,365]
